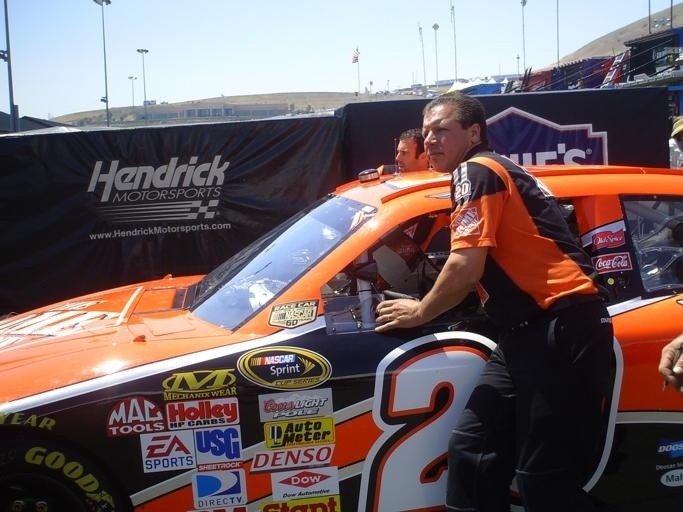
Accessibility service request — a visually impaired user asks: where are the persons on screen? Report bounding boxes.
[392,127,451,296]
[656,333,683,395]
[375,95,615,512]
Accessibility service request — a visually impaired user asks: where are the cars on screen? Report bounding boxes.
[0,163,683,511]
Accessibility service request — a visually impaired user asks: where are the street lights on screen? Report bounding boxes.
[94,0,112,126]
[432,24,439,81]
[138,49,148,126]
[128,76,137,122]
[521,1,527,72]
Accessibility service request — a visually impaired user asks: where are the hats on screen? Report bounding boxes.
[671,120,683,137]
